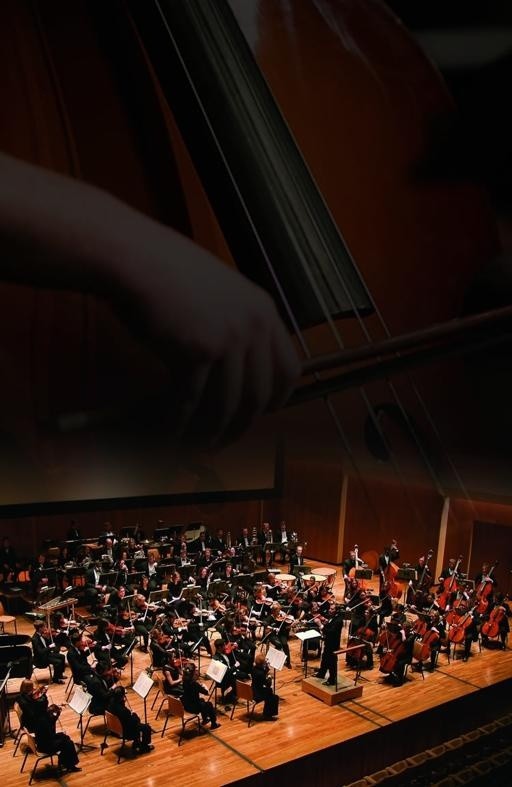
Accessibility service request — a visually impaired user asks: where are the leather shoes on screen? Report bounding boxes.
[63,764,82,770]
[502,642,505,648]
[312,674,325,678]
[140,745,154,751]
[206,717,220,729]
[322,681,336,685]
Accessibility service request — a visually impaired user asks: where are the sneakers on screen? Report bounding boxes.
[463,655,468,661]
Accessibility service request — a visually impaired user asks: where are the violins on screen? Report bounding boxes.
[31,583,337,719]
[364,598,376,611]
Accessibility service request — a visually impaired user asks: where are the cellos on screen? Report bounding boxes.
[347,544,508,673]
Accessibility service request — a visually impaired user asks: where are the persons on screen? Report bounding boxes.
[0,519,511,775]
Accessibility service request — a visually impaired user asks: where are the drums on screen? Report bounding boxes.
[275,574,296,586]
[301,574,327,593]
[311,568,336,585]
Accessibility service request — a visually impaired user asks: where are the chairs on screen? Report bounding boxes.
[0,526,512,787]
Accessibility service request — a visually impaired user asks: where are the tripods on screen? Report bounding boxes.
[3,528,369,757]
